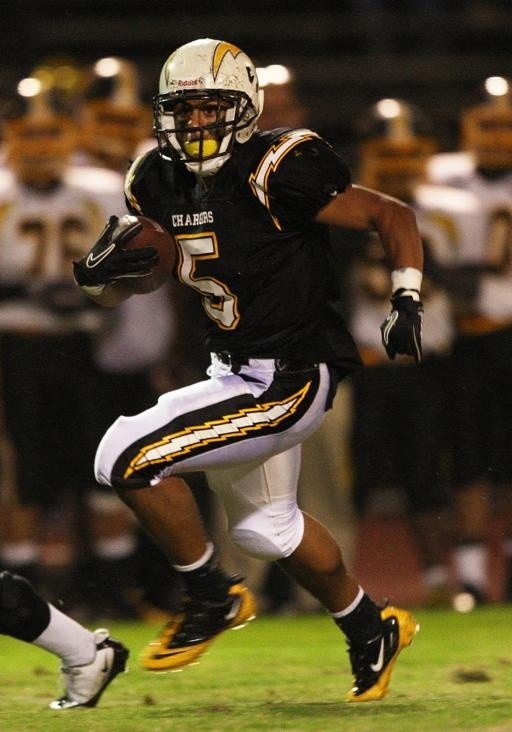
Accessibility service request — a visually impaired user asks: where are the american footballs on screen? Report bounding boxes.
[111,212,175,293]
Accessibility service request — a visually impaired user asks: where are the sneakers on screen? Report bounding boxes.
[45,628,133,712]
[136,579,261,677]
[341,595,421,706]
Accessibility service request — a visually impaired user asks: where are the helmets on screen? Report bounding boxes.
[352,69,512,175]
[147,35,269,179]
[2,54,146,137]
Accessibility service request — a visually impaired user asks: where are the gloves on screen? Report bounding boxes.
[376,286,424,366]
[70,214,158,290]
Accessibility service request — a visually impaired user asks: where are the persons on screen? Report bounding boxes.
[1,572,132,715]
[70,34,425,704]
